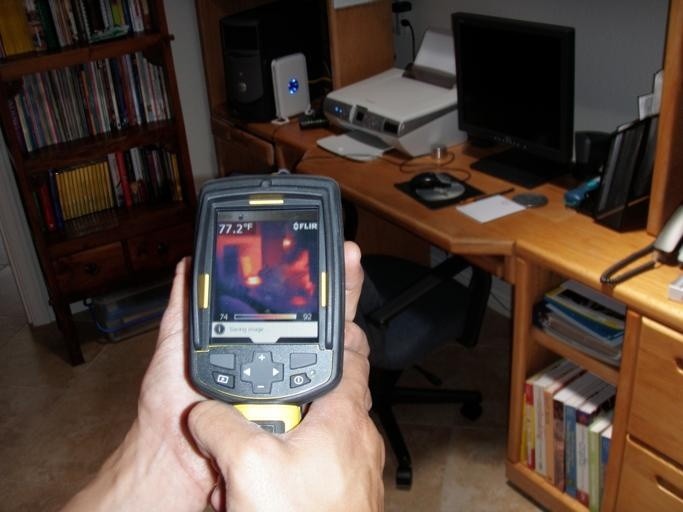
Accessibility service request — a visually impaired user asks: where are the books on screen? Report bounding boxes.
[8,51,171,151]
[26,0,152,51]
[521,277,632,512]
[30,142,182,230]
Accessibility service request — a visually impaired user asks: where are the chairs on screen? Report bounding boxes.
[355,256,487,492]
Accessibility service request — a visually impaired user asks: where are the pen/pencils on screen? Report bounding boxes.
[462,188,514,203]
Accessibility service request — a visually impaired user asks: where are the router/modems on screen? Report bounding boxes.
[271,52,315,125]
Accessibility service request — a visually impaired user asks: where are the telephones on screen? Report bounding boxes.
[641,202,683,265]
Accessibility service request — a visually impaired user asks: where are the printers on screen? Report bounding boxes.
[322,27,467,157]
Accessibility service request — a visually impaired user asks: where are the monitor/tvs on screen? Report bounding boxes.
[450,12,575,187]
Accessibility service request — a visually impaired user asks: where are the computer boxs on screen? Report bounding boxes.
[219,0,331,121]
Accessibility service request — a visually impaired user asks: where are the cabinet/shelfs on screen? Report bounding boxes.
[0,0,201,368]
[193,1,683,511]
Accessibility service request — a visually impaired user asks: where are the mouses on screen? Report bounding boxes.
[411,170,437,190]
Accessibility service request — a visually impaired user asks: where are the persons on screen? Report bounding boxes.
[63,240,385,512]
